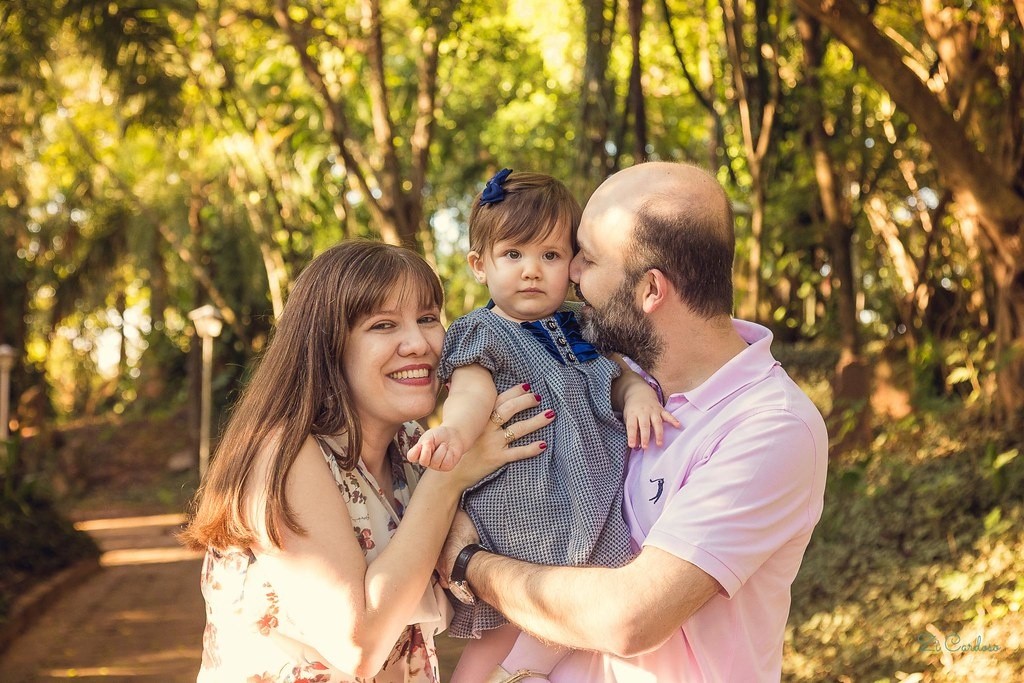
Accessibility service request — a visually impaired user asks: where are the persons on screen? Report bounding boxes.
[182,240,556,682]
[433,162,829,683]
[407,169,681,683]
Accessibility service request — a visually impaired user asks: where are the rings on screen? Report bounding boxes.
[503,427,516,444]
[490,410,505,428]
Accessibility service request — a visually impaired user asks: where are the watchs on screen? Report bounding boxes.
[448,543,492,607]
